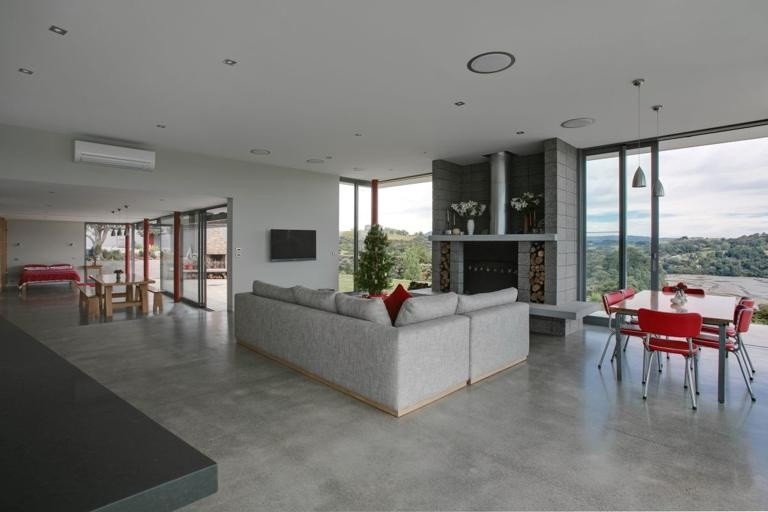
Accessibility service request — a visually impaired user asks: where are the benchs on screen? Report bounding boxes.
[74,274,166,317]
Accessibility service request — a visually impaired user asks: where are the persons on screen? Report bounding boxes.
[186,243,195,279]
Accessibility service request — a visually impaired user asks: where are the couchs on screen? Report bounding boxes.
[232,279,530,418]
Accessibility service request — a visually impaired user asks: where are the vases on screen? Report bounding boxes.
[466,218,475,235]
[522,214,530,233]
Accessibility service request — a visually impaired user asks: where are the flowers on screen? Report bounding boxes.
[114,269,123,273]
[452,199,486,218]
[508,190,543,214]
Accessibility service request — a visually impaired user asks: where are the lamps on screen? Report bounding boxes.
[109,227,129,237]
[630,77,647,190]
[650,103,666,198]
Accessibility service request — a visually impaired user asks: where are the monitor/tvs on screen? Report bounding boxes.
[270,228,316,262]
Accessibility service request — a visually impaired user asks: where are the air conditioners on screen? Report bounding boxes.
[70,138,159,172]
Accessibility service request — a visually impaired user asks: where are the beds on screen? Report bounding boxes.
[19,262,80,297]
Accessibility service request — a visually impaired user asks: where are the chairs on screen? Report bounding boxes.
[594,278,761,410]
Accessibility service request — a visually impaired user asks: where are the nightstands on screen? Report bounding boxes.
[81,264,103,281]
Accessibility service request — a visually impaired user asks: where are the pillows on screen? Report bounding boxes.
[23,265,74,271]
[384,283,413,325]
